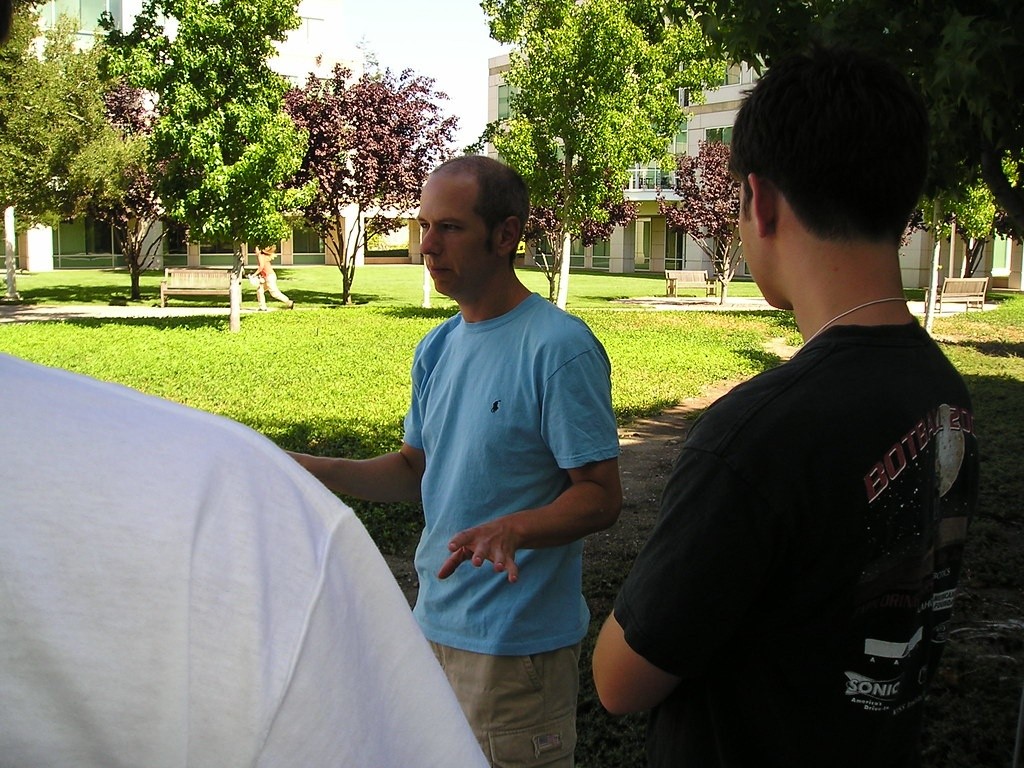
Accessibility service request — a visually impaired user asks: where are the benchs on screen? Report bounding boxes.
[664,269,718,298]
[158,268,232,308]
[924,277,990,314]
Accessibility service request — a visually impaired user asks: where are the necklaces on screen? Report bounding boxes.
[784,295,908,361]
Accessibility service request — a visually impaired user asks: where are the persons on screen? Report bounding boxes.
[2,346,490,766]
[249,245,295,312]
[590,38,980,768]
[277,156,624,767]
